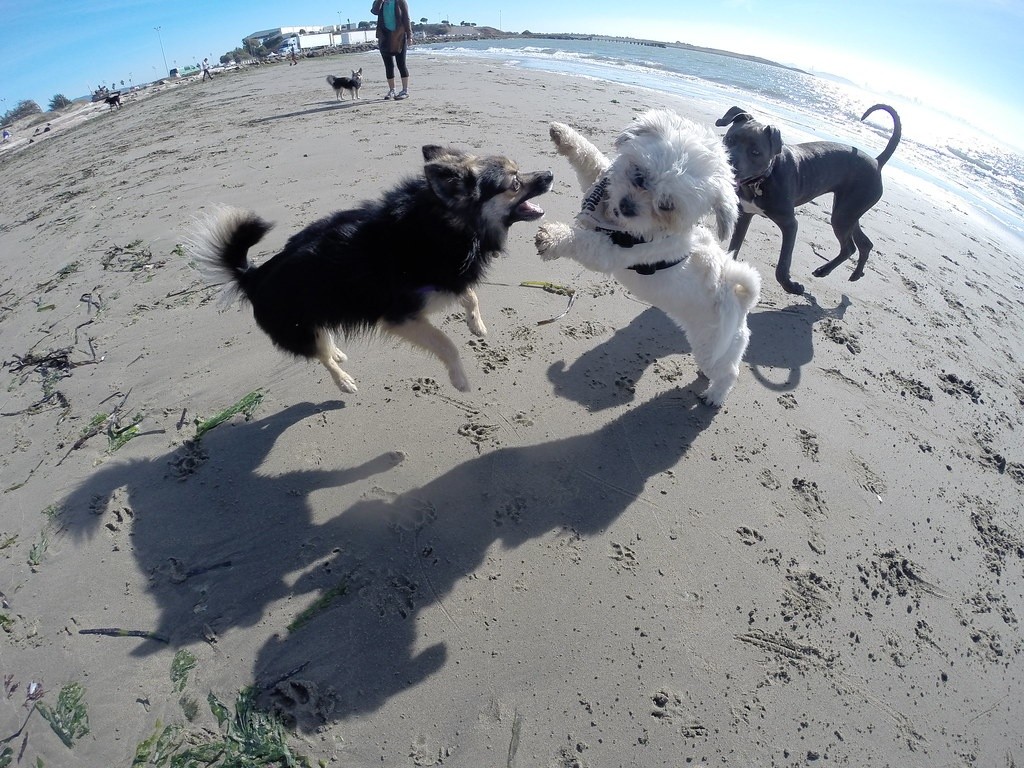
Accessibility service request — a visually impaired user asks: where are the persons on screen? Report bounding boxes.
[370,0,412,100]
[290,43,297,66]
[202,58,213,83]
[3,130,9,140]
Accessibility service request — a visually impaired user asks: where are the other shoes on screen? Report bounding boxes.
[395,90,409,100]
[385,90,398,100]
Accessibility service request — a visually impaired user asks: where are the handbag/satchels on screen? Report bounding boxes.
[380,25,404,54]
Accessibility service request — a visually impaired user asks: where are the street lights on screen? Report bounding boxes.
[154,26,169,76]
[338,11,343,25]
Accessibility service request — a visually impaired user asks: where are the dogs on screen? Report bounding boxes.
[189,103,902,410]
[326,67,363,101]
[104,91,122,111]
